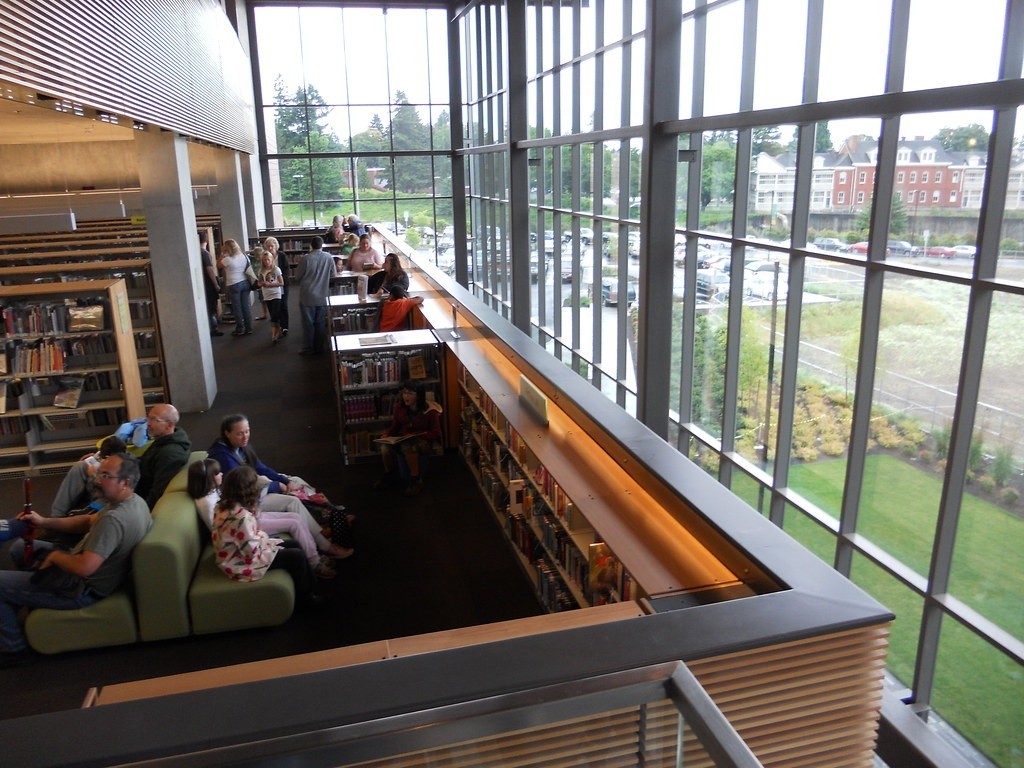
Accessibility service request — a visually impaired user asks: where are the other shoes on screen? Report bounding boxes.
[255,316,267,320]
[299,350,324,357]
[315,503,354,578]
[276,333,282,339]
[210,330,224,337]
[231,330,244,335]
[245,330,252,334]
[382,472,393,486]
[0,647,28,671]
[405,478,425,497]
[272,336,277,342]
[280,327,288,334]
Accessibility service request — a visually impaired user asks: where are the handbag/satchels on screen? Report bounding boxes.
[244,254,259,287]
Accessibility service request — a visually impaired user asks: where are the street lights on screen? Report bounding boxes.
[293,174,305,226]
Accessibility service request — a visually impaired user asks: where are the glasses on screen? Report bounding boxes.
[95,472,122,480]
[149,417,171,423]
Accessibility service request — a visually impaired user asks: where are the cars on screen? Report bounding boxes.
[951,245,978,261]
[380,220,957,303]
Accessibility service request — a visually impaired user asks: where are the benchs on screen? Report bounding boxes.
[27,452,296,654]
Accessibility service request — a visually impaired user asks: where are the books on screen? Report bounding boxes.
[454,364,638,613]
[328,284,376,332]
[250,240,306,277]
[338,349,427,458]
[0,256,165,434]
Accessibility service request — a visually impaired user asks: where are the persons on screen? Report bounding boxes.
[373,380,444,494]
[254,236,290,341]
[189,415,356,582]
[375,252,409,294]
[328,214,379,273]
[295,235,337,356]
[0,402,191,668]
[217,240,254,335]
[378,286,423,333]
[199,232,224,336]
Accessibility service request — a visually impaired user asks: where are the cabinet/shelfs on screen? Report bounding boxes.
[259,232,312,281]
[323,243,343,254]
[324,295,415,334]
[332,253,350,264]
[332,343,445,467]
[445,354,644,611]
[330,270,381,292]
[0,214,222,454]
